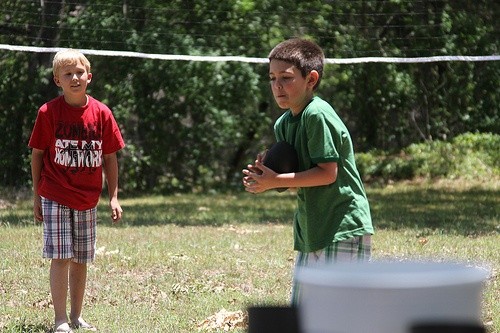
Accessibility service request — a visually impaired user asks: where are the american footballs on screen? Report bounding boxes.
[263,141,301,193]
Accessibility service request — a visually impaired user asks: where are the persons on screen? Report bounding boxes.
[28,49,126,333]
[242,38,375,306]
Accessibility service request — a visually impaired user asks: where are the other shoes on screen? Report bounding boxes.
[55,324,72,333]
[72,319,97,331]
